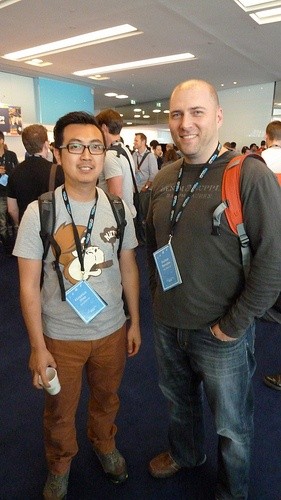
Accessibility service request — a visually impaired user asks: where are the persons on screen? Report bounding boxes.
[0,110,281,389]
[146,81,281,500]
[12,109,142,500]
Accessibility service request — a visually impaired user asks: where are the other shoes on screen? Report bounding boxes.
[263,374,281,390]
[147,451,207,479]
[95,446,128,484]
[43,460,70,500]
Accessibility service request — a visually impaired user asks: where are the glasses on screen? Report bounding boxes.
[58,143,106,155]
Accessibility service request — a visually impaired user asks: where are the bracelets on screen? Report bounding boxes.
[145,185,149,189]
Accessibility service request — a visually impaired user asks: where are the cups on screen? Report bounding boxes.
[39,367,61,395]
